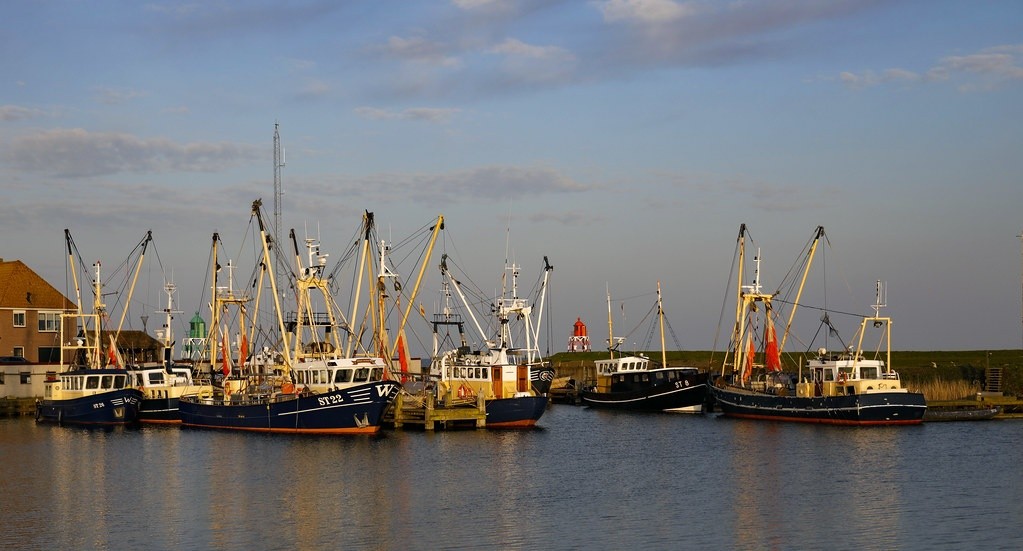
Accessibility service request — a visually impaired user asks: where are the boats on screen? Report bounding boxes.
[579,282,710,417]
[34,197,557,432]
[704,222,929,428]
[550,375,577,406]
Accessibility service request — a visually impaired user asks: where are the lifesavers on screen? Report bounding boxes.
[294,388,303,399]
[836,372,847,385]
[457,384,469,400]
[224,382,232,396]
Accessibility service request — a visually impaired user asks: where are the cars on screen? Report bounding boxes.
[0,356,30,363]
